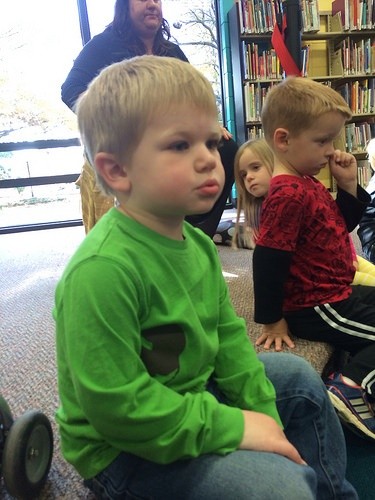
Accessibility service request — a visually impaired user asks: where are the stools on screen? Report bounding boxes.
[73,159,116,237]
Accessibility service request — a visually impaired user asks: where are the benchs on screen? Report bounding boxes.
[224,267,334,380]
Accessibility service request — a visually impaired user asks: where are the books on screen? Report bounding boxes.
[237,0,375,192]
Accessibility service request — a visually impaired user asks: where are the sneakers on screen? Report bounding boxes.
[325,370,375,441]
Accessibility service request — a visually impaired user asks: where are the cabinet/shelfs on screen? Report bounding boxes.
[215,0,375,209]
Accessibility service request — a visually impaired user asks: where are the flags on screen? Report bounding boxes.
[271,0,309,77]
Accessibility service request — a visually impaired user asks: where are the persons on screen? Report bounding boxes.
[356,138,375,266]
[252,75,375,442]
[61,0,240,240]
[51,53,360,500]
[232,138,375,287]
